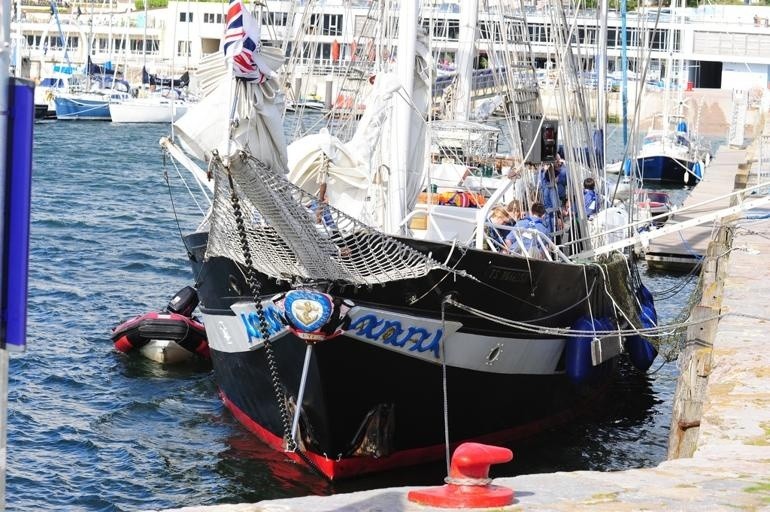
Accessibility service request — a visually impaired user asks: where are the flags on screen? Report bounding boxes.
[224,0,265,84]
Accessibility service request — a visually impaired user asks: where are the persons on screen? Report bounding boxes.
[484,157,600,260]
